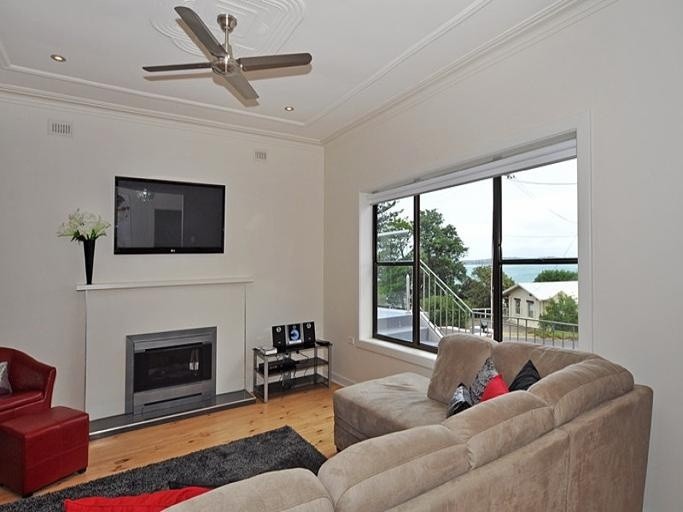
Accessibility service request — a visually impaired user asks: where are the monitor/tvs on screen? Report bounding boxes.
[114,177,225,253]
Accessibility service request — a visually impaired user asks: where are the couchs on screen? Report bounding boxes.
[162,332,654,512]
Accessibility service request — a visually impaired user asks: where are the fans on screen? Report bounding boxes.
[142,6,313,102]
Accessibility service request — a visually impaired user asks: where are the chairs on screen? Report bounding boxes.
[1,347,57,424]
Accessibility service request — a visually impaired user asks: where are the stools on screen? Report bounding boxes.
[1,405,90,498]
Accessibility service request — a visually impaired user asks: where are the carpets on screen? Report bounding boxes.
[0,423,332,512]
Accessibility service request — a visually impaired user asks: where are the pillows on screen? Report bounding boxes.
[446,355,541,416]
[63,486,213,511]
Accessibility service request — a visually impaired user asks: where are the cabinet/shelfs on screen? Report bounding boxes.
[253,340,333,404]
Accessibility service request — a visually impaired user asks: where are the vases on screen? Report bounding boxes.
[80,232,97,284]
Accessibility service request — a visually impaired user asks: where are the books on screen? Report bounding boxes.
[256,344,278,356]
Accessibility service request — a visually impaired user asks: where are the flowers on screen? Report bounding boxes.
[57,209,114,241]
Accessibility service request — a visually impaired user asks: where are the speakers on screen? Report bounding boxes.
[272,324,286,353]
[303,320,315,349]
